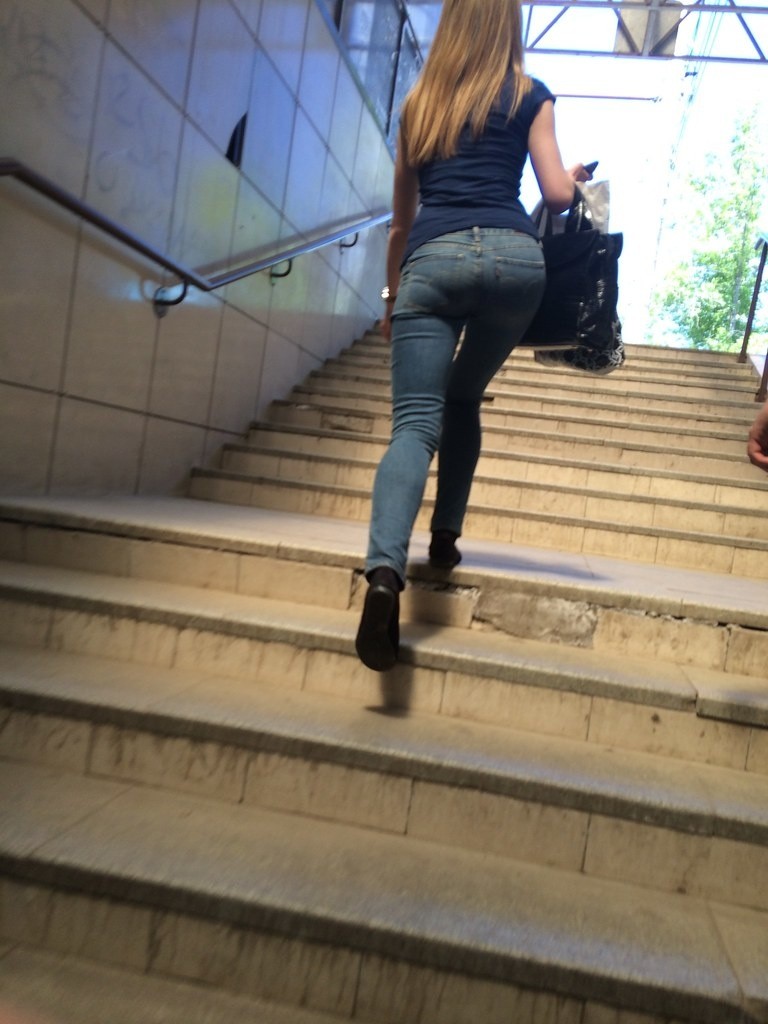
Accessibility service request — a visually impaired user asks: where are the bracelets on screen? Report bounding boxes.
[385,296,397,304]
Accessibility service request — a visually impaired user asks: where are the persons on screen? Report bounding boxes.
[353,0,593,674]
[748,354,768,470]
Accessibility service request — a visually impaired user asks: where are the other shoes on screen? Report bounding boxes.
[356,568,401,670]
[429,531,462,571]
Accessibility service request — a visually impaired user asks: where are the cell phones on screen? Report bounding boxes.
[582,161,599,174]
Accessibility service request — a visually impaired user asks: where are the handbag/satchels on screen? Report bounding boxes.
[532,180,622,377]
[521,179,611,353]
